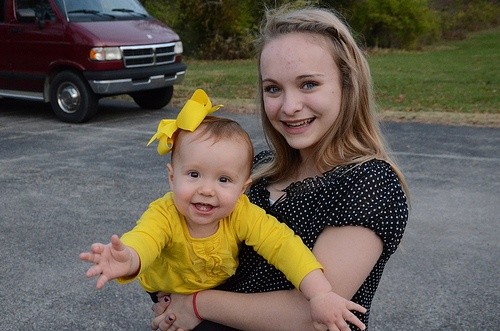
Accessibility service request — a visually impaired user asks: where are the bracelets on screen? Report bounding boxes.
[193,292,204,322]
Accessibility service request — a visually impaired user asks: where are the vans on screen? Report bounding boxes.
[0,0,187,124]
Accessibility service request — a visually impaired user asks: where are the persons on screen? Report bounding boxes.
[151,9,409,331]
[80,89,366,331]
